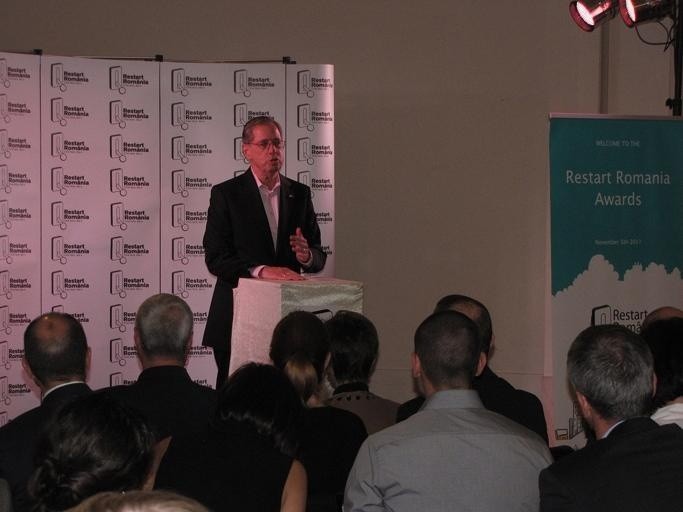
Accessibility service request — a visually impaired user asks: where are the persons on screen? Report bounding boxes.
[202,116,327,389]
[539,306,683,511]
[1,294,404,510]
[342,295,554,511]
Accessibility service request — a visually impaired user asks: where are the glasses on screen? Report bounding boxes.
[245,139,286,150]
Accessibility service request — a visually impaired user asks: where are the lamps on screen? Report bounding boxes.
[568,0,619,33]
[612,0,679,28]
